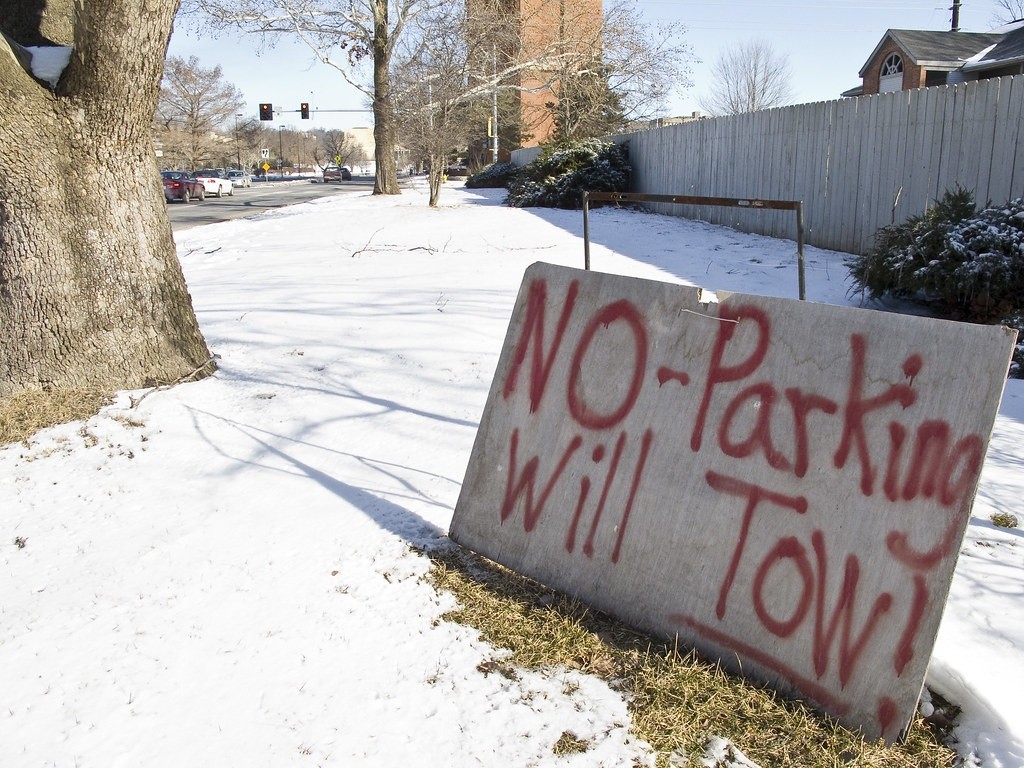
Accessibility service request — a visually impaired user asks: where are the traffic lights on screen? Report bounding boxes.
[259,103,273,121]
[300,102,309,119]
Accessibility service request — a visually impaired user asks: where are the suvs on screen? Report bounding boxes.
[159,170,206,205]
[191,170,235,198]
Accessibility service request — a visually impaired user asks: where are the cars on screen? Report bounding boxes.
[215,167,232,177]
[226,170,251,188]
[323,167,351,183]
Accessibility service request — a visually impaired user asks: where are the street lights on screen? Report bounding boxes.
[279,125,285,175]
[235,114,243,170]
